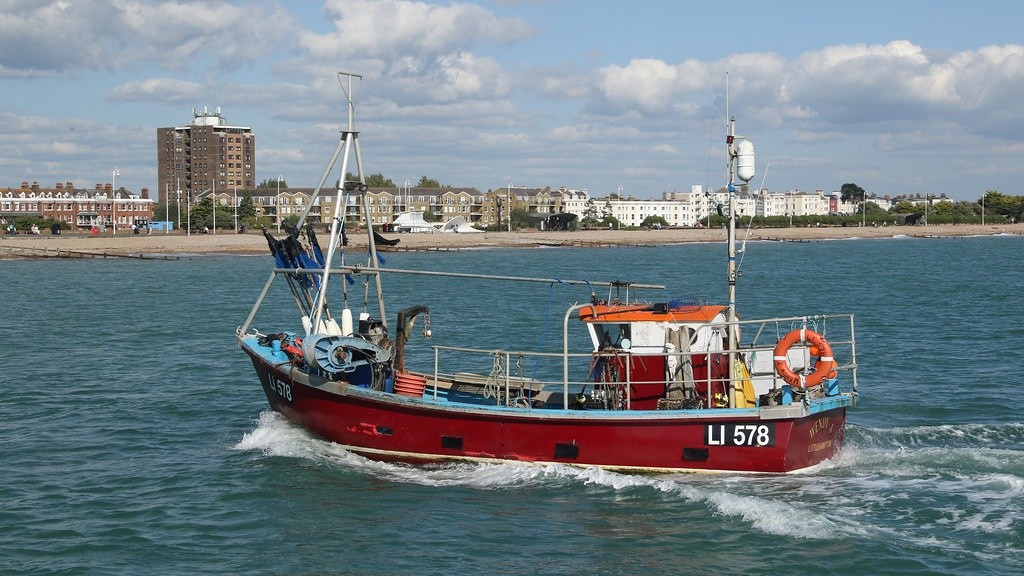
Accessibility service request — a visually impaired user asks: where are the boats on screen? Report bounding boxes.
[235,68,861,471]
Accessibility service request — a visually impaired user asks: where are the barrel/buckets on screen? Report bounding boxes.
[393,370,427,398]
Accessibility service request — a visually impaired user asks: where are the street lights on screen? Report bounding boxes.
[981,193,987,226]
[924,192,930,228]
[276,174,284,234]
[507,183,513,232]
[404,180,411,213]
[863,191,869,227]
[789,190,796,228]
[617,185,623,230]
[112,167,120,236]
[707,187,713,228]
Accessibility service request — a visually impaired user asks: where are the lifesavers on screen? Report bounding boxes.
[772,329,834,388]
